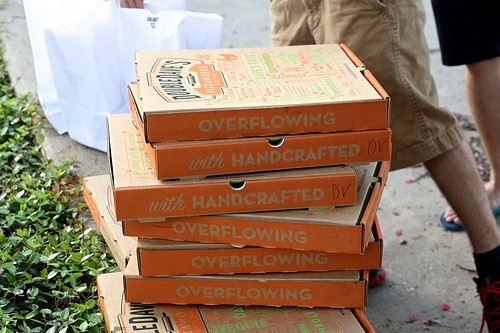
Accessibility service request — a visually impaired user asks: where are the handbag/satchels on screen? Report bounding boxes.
[24,0,222,152]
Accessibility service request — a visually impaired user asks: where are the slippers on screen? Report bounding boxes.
[441,204,500,230]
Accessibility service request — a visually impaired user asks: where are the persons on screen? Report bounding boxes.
[118,0,500,333]
[430,0,500,232]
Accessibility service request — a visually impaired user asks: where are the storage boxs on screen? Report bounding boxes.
[81,40,395,333]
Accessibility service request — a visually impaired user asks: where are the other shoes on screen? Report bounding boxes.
[361,268,386,286]
[472,279,499,333]
